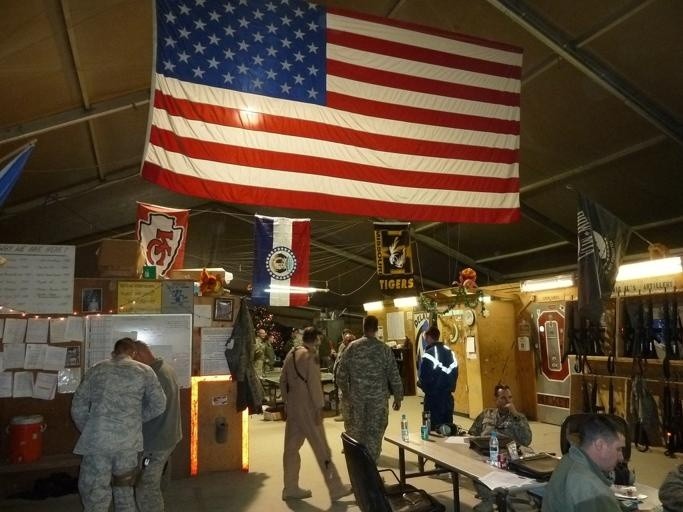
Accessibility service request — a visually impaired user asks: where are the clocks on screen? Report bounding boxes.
[464,309,474,326]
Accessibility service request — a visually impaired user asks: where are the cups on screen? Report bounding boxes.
[387,341,403,349]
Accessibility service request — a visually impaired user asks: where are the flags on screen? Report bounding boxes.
[252,213,311,307]
[140,0,523,225]
[575,188,634,321]
[134,202,188,279]
[374,221,416,291]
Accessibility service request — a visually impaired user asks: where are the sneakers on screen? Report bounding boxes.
[325,483,355,502]
[280,487,313,502]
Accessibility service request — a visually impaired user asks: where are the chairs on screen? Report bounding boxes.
[560,414,632,464]
[341,432,444,511]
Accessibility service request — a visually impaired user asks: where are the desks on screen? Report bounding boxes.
[384,424,563,511]
[264,367,339,418]
[529,481,664,512]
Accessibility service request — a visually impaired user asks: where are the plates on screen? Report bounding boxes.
[613,492,647,500]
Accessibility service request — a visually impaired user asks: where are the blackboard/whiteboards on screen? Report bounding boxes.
[84,314,192,389]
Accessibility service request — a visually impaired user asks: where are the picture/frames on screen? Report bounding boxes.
[82,287,103,313]
[213,297,235,322]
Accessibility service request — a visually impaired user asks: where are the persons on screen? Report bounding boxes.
[468,384,532,512]
[134,340,184,512]
[333,314,404,480]
[87,297,100,312]
[69,336,168,512]
[255,327,357,422]
[416,325,459,469]
[657,463,683,512]
[279,326,354,501]
[539,416,627,512]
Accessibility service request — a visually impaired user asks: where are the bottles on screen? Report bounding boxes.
[401,414,408,442]
[489,431,510,471]
[421,411,431,435]
